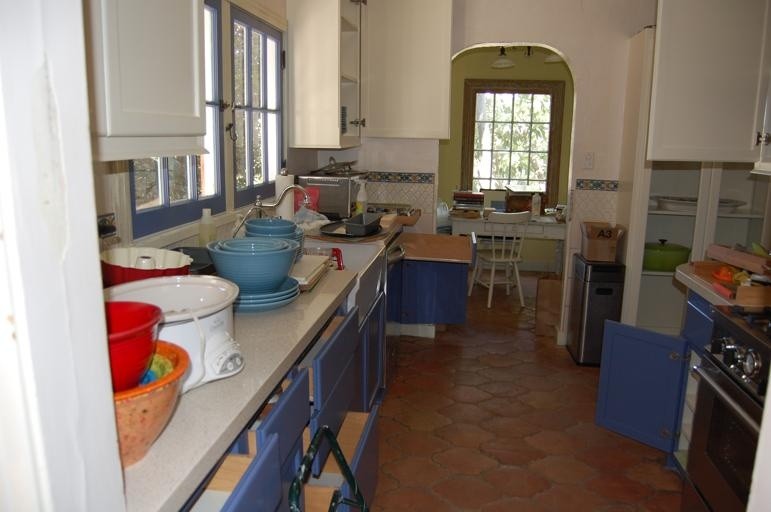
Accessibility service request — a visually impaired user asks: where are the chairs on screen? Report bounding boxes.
[467,210,531,309]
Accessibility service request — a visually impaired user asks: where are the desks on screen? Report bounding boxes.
[447,215,566,241]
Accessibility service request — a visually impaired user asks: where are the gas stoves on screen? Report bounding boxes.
[712,303,770,349]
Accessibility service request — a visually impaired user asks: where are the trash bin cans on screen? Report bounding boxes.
[565,252,626,368]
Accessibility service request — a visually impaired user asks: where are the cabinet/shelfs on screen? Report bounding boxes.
[595,286,716,481]
[286,0,454,150]
[147,227,480,512]
[617,25,771,337]
[84,0,210,160]
[645,0,771,178]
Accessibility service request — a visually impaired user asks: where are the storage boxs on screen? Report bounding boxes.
[580,219,626,263]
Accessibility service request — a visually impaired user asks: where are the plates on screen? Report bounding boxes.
[650,194,747,215]
[245,216,303,266]
[231,276,301,313]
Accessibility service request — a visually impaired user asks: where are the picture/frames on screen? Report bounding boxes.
[479,188,506,213]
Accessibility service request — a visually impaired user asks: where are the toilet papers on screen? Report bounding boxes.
[275,174,295,221]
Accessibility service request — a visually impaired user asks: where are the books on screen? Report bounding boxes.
[453,191,485,211]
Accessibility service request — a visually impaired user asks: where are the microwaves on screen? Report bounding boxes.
[296,174,368,220]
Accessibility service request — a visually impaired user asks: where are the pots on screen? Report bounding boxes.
[642,238,692,272]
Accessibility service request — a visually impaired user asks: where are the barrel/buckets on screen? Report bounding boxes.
[437,198,449,227]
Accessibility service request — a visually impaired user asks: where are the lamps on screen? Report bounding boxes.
[543,54,563,63]
[491,46,515,69]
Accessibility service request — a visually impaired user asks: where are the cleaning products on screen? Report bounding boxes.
[354,179,368,215]
[532,193,541,216]
[198,208,215,246]
[231,213,246,239]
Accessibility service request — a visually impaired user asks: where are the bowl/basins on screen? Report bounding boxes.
[204,237,307,291]
[114,340,190,471]
[104,300,162,394]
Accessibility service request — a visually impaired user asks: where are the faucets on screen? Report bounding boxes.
[254,184,312,218]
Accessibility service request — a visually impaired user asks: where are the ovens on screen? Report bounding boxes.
[683,353,763,512]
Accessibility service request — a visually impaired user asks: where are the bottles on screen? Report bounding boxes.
[532,192,541,220]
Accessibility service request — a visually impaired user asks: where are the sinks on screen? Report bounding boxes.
[300,235,388,326]
